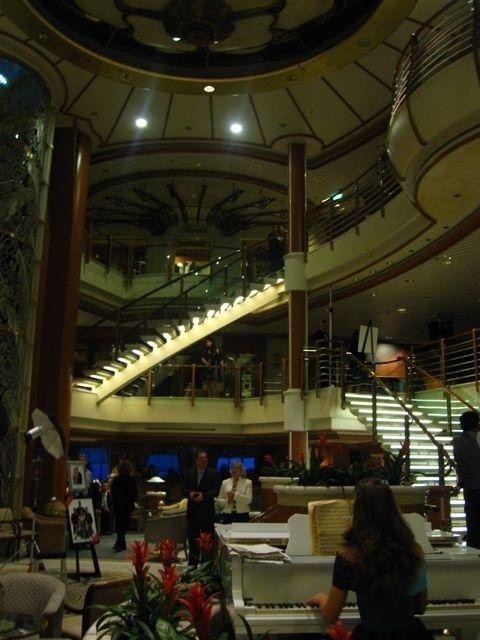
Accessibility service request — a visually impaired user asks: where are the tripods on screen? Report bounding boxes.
[0,453,56,572]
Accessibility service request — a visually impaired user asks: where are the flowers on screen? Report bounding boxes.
[261,452,300,476]
[322,621,353,639]
[290,431,425,486]
[86,530,230,640]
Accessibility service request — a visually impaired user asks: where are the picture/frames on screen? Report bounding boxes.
[63,494,101,546]
[66,459,90,492]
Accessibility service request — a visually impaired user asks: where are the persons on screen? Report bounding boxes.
[93,340,114,370]
[78,448,253,566]
[348,329,366,393]
[201,337,224,397]
[268,223,285,272]
[453,411,480,549]
[304,476,436,640]
[314,320,335,387]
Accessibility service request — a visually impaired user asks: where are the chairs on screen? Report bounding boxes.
[60,576,153,639]
[0,507,17,562]
[1,572,66,639]
[20,505,68,559]
[1,507,40,562]
[144,498,190,561]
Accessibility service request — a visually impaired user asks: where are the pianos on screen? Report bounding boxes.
[214,523,480,640]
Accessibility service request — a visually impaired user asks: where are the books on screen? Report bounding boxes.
[308,499,357,556]
